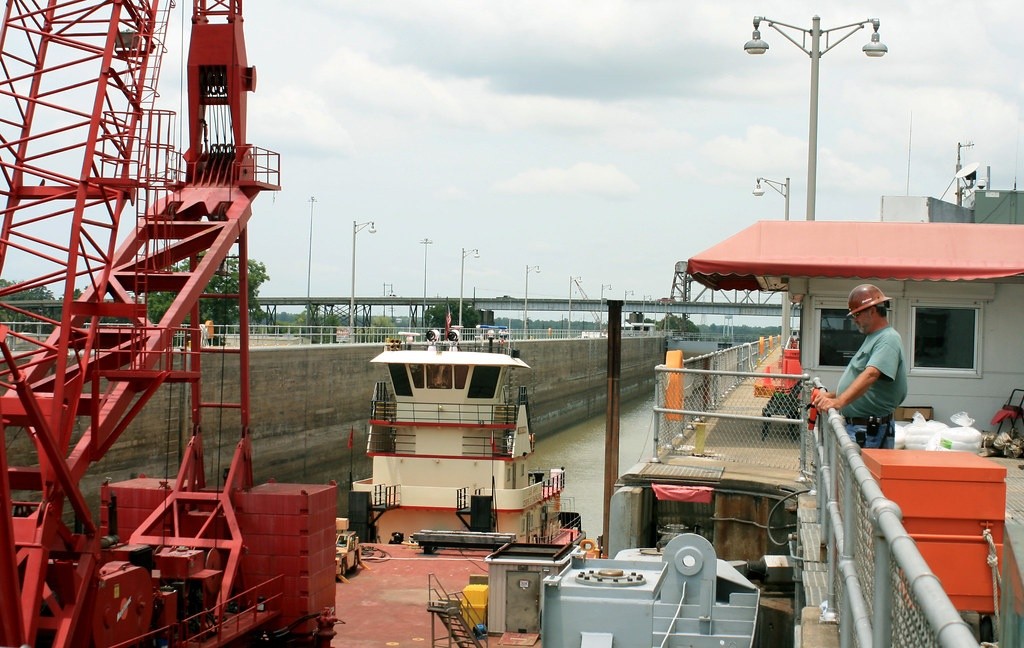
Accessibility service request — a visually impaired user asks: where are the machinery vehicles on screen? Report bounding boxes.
[0,2,346,648]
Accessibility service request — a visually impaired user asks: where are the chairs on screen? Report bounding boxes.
[995,387,1024,432]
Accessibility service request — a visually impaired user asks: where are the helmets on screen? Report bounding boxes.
[847,284,892,316]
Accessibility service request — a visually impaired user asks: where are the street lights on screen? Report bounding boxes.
[418,238,434,327]
[600,284,612,338]
[568,276,583,338]
[458,246,481,338]
[523,264,540,339]
[623,290,633,331]
[754,176,790,347]
[641,293,673,333]
[349,220,376,341]
[742,16,888,220]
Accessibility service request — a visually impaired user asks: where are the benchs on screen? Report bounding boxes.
[412,528,516,556]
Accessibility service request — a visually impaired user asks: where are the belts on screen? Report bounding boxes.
[845,413,892,425]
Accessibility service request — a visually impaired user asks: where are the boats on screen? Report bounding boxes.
[347,330,587,545]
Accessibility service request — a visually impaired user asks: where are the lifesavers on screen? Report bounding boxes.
[579,540,595,552]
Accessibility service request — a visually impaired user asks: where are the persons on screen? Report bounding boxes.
[205,317,216,346]
[811,284,909,448]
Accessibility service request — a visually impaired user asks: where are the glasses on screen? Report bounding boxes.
[852,312,860,318]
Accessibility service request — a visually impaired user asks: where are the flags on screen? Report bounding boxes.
[444,297,451,339]
